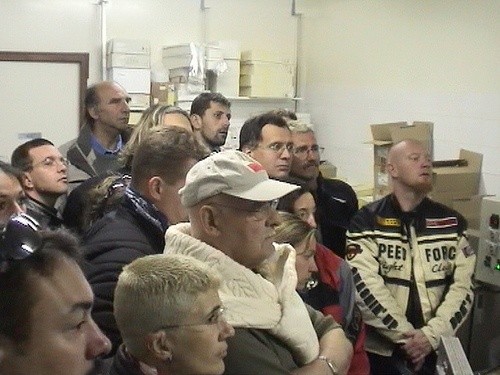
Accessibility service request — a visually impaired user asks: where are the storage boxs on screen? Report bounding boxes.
[368,119,434,202]
[205,45,240,96]
[240,50,293,99]
[106,39,205,125]
[432,148,484,230]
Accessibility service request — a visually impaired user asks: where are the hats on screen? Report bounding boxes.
[178,150,301,208]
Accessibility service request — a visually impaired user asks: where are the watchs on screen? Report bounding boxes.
[316,354,339,375]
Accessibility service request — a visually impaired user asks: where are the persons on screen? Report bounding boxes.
[345,139,476,374]
[162,150,355,374]
[0,213,113,375]
[0,81,372,375]
[109,253,236,374]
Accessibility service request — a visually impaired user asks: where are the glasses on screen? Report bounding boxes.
[33,156,70,169]
[0,213,43,272]
[207,201,278,220]
[257,142,294,153]
[153,306,222,333]
[294,144,324,156]
